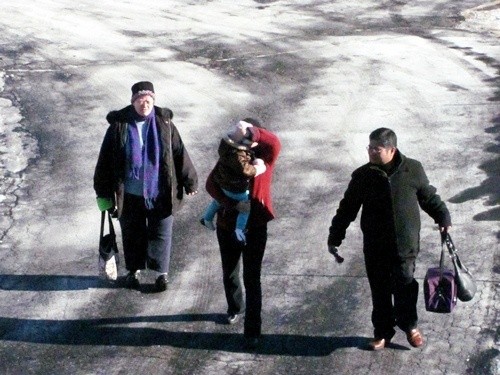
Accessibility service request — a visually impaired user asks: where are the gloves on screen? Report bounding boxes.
[253,158,267,177]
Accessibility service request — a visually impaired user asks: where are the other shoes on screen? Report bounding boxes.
[244,334,261,349]
[369,335,392,351]
[235,228,247,242]
[225,312,240,325]
[155,274,169,290]
[396,322,423,348]
[200,218,215,231]
[126,269,141,285]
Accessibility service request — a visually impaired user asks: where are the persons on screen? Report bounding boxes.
[94,82,199,290]
[328,128,452,352]
[205,117,282,350]
[198,119,267,245]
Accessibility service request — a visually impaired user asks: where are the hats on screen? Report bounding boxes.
[223,119,258,151]
[131,81,155,104]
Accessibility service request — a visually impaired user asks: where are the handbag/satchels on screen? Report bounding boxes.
[445,232,476,302]
[98,209,120,285]
[423,230,456,313]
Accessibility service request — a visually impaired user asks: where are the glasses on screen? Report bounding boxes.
[366,145,382,153]
